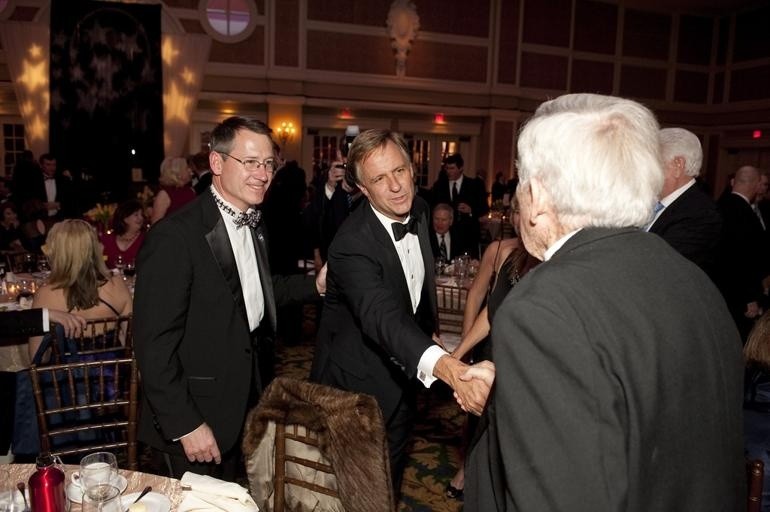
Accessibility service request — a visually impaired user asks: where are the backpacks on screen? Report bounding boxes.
[12,322,123,460]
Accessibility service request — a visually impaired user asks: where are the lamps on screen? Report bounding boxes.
[276,122,296,145]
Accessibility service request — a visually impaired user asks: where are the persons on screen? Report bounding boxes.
[39,156,66,225]
[717,166,769,410]
[1,178,12,200]
[475,167,488,191]
[261,140,308,276]
[309,129,491,511]
[12,150,49,254]
[444,197,520,497]
[150,155,197,226]
[193,152,213,194]
[132,115,328,481]
[642,129,752,348]
[130,181,150,209]
[750,171,769,234]
[30,219,133,408]
[0,201,25,262]
[0,307,88,456]
[743,275,770,512]
[451,241,543,360]
[99,202,146,272]
[492,171,504,204]
[429,204,486,260]
[465,94,750,511]
[311,132,364,265]
[504,168,518,207]
[416,154,490,260]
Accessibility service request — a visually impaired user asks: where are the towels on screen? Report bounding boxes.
[180,470,259,512]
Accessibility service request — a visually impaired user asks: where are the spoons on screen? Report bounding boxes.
[17,481,30,511]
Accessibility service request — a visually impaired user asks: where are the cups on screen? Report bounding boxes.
[17,282,24,291]
[461,256,470,267]
[24,254,36,278]
[71,462,111,494]
[124,264,135,284]
[115,256,125,275]
[6,283,16,300]
[435,260,444,280]
[80,452,119,511]
[0,469,13,511]
[30,281,36,293]
[6,272,14,282]
[454,257,464,282]
[38,260,48,280]
[81,484,122,512]
[467,264,475,280]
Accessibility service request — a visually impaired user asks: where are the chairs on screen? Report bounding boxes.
[26,344,143,474]
[26,309,137,439]
[433,284,473,338]
[274,424,340,512]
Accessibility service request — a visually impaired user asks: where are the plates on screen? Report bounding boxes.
[14,487,71,511]
[99,492,172,512]
[66,474,128,505]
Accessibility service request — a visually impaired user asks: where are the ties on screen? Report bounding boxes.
[440,234,447,264]
[452,182,457,201]
[652,200,664,212]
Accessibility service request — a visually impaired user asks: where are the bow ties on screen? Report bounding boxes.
[210,190,262,229]
[44,176,55,180]
[391,220,418,241]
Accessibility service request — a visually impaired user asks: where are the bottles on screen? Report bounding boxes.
[27,453,64,512]
